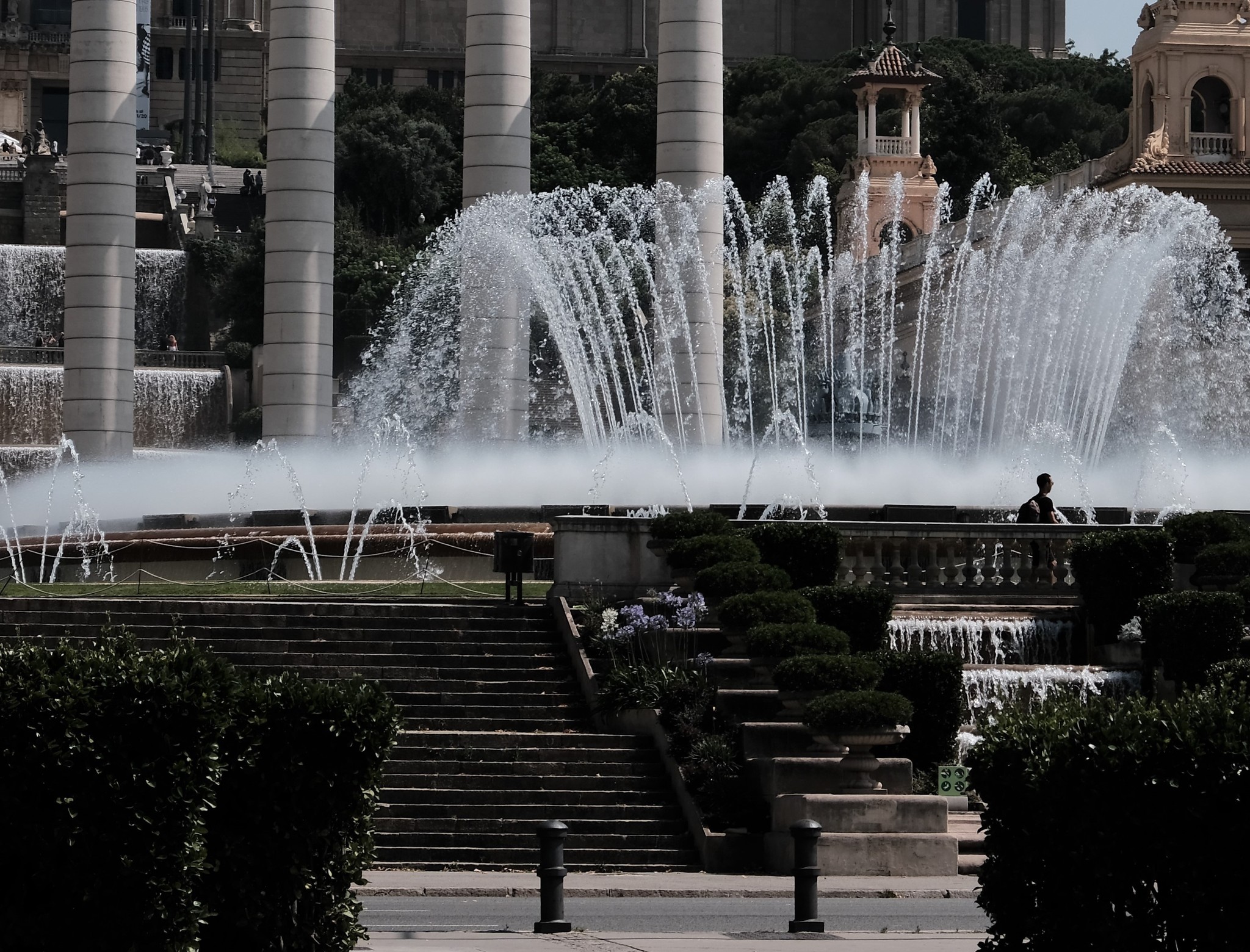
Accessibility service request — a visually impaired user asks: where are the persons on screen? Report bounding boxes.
[32,119,51,155]
[234,225,242,241]
[243,169,263,195]
[1027,473,1060,586]
[158,334,178,361]
[0,130,59,161]
[135,144,155,165]
[35,332,64,365]
[213,225,220,241]
[198,175,210,210]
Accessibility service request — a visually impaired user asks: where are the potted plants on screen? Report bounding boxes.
[745,622,851,690]
[774,655,885,753]
[651,511,733,595]
[1195,540,1250,591]
[803,690,912,795]
[714,590,818,659]
[667,535,762,606]
[696,560,793,623]
